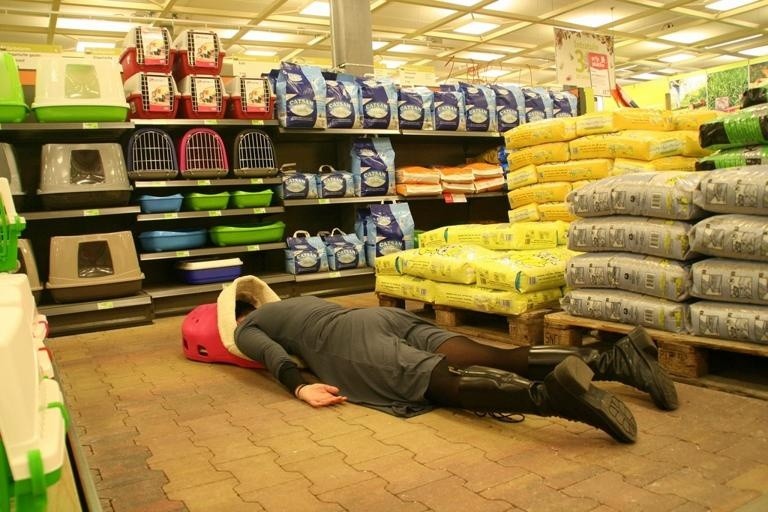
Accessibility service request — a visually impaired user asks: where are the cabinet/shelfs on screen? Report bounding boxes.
[0,70,154,338]
[402,87,585,247]
[130,77,296,321]
[275,71,401,298]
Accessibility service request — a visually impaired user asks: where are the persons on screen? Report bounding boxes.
[233,294,679,443]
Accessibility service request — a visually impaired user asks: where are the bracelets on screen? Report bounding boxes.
[296,384,306,401]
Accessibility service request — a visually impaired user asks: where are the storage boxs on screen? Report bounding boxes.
[32,57,130,122]
[230,78,277,120]
[0,54,32,122]
[119,27,174,72]
[36,142,133,206]
[179,79,230,118]
[0,239,43,305]
[171,258,243,285]
[46,231,144,303]
[0,144,27,212]
[124,73,180,120]
[173,29,224,76]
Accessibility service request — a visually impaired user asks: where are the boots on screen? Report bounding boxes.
[524,325,678,410]
[447,355,637,443]
